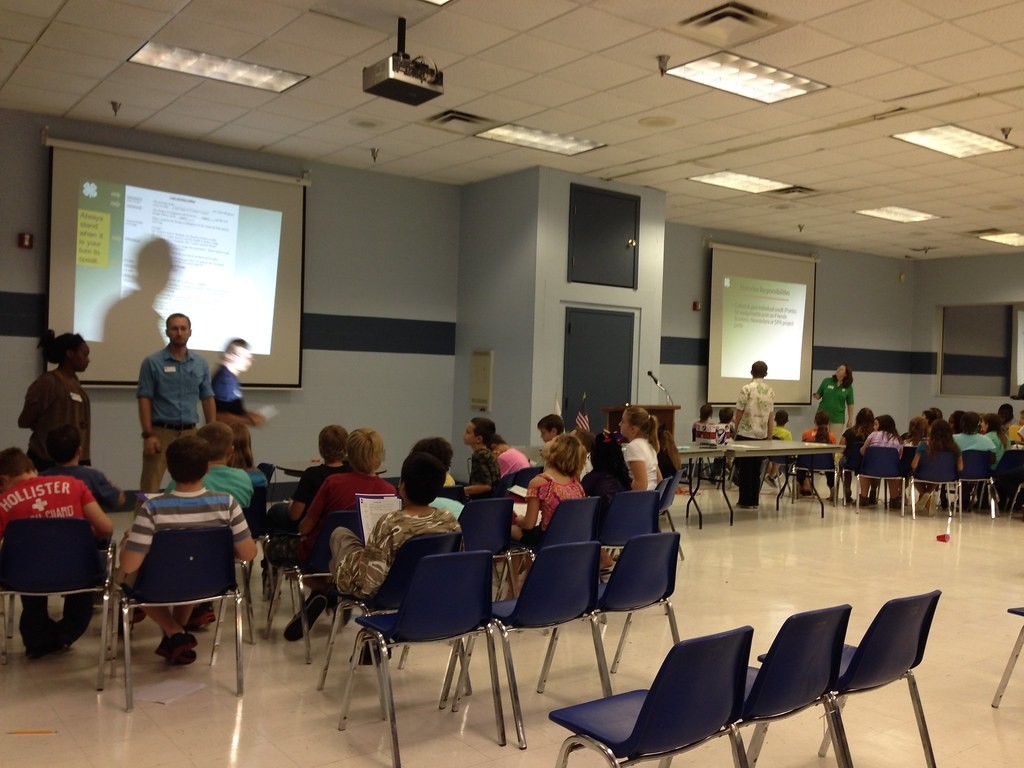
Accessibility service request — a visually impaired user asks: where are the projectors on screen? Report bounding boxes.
[363,56,444,106]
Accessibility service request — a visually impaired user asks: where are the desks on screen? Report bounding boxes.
[277,460,387,477]
[686,439,846,526]
[622,442,724,529]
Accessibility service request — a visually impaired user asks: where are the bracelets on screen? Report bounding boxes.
[141,431,155,438]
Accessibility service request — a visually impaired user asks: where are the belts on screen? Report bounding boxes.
[153,422,196,429]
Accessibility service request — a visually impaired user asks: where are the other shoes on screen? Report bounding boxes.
[800,488,811,496]
[829,487,834,502]
[132,607,147,624]
[284,592,327,641]
[736,503,759,509]
[350,641,392,665]
[869,497,878,504]
[184,604,216,628]
[973,501,988,510]
[889,497,908,508]
[859,494,869,505]
[842,496,853,501]
[25,643,70,658]
[951,502,964,511]
[915,492,937,517]
[765,476,778,488]
[333,604,352,627]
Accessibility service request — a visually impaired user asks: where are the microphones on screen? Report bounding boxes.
[647,370,659,385]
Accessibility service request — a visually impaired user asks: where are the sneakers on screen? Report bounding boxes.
[154,633,198,663]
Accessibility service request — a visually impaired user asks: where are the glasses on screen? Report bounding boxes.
[232,353,253,361]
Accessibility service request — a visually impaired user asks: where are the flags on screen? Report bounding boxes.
[575,398,591,433]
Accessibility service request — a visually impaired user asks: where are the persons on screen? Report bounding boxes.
[692,403,1024,517]
[850,414,907,509]
[796,411,838,502]
[910,419,963,518]
[327,451,466,665]
[734,360,775,509]
[0,446,114,659]
[211,339,266,426]
[133,312,217,521]
[812,363,854,476]
[764,409,794,489]
[0,406,681,667]
[511,432,587,551]
[118,433,257,667]
[18,328,92,473]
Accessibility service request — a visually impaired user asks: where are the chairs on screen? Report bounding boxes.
[0,428,1024,768]
